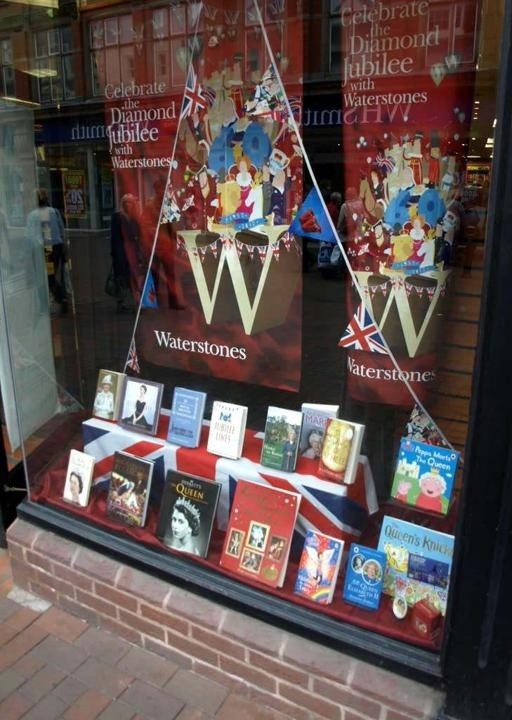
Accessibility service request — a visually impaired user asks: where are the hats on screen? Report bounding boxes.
[102,375,113,386]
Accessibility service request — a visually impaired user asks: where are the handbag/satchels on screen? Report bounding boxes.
[330,246,343,269]
[317,242,332,268]
[105,276,128,299]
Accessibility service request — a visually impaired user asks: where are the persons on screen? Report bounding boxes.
[69,471,83,504]
[454,173,489,277]
[230,524,284,570]
[354,556,364,571]
[302,177,365,275]
[311,552,324,585]
[364,562,380,584]
[281,429,297,472]
[93,374,113,419]
[16,188,72,316]
[123,385,147,426]
[107,480,139,521]
[110,193,151,313]
[141,178,192,310]
[171,498,201,557]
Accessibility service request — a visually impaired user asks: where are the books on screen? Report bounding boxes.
[390,437,462,516]
[375,516,456,618]
[316,417,366,485]
[63,449,95,507]
[290,529,346,606]
[207,400,248,459]
[92,369,127,423]
[105,449,155,528]
[259,405,304,472]
[299,402,340,460]
[220,479,302,588]
[165,387,207,448]
[341,543,387,612]
[152,469,222,559]
